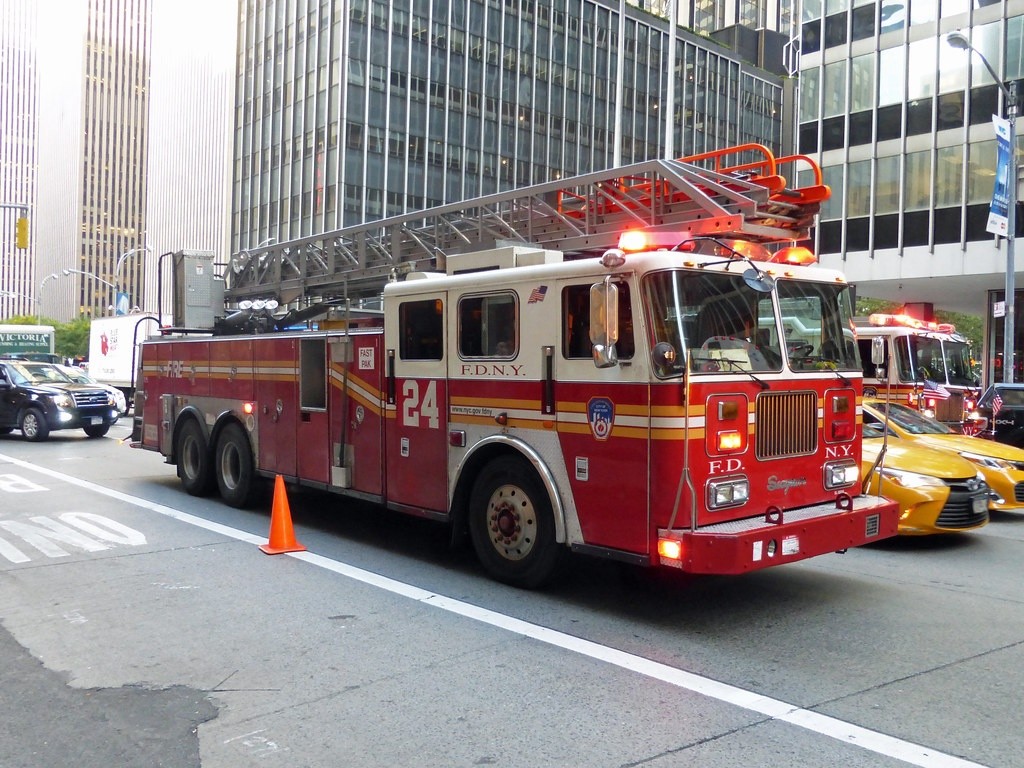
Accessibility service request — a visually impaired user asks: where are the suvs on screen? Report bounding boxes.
[0,359,115,441]
[972,383,1024,450]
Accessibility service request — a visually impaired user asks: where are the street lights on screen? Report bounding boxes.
[1,274,59,325]
[946,30,1018,385]
[108,305,141,316]
[62,246,152,316]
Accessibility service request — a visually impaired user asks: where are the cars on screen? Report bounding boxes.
[41,363,127,425]
[862,422,991,534]
[862,397,1024,509]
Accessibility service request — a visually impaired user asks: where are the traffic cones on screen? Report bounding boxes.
[259,473,309,554]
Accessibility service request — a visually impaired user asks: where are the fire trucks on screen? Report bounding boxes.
[129,143,901,587]
[664,312,988,437]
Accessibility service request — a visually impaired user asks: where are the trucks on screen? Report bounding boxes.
[89,311,214,417]
